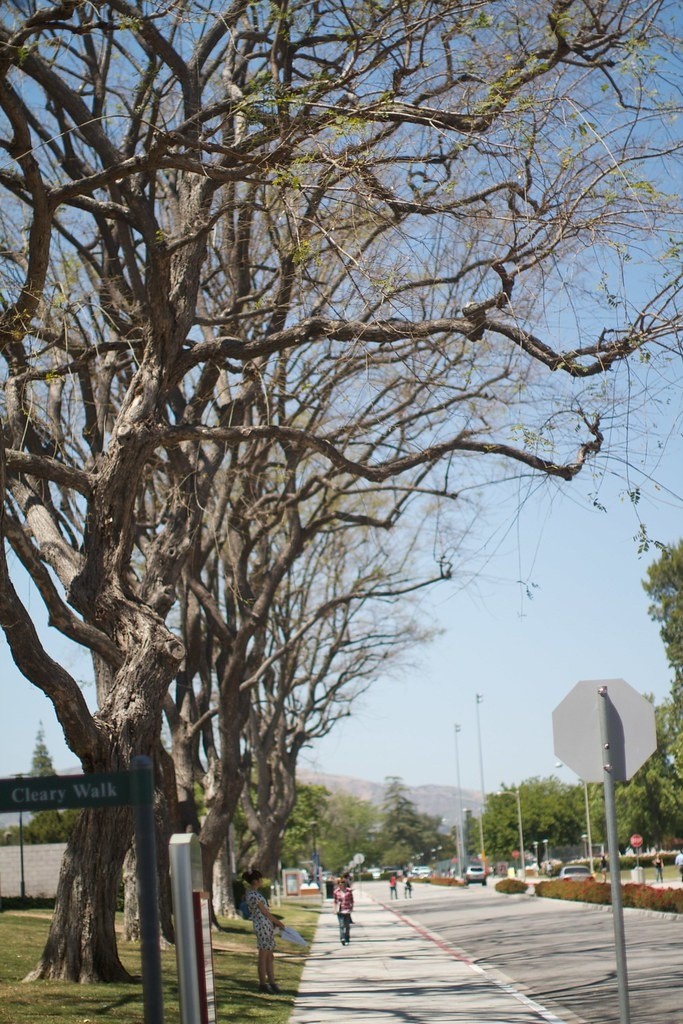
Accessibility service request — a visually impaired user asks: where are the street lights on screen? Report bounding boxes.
[311,821,319,879]
[554,763,596,876]
[581,832,589,859]
[464,806,487,862]
[475,692,486,804]
[495,787,526,883]
[11,770,33,901]
[532,840,539,862]
[541,839,550,863]
[455,722,464,854]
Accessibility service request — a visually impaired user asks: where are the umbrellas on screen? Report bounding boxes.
[278,921,311,949]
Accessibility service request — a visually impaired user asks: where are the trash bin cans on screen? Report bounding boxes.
[325,880,334,898]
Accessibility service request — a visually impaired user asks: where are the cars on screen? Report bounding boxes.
[552,865,597,882]
[366,866,431,879]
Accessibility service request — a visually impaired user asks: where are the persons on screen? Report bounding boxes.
[447,850,683,883]
[333,867,412,947]
[243,870,280,992]
[235,882,252,919]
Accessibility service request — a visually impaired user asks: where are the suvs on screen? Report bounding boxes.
[463,866,487,886]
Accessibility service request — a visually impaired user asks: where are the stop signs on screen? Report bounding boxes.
[631,834,643,847]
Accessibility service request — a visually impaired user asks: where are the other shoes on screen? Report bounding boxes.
[341,939,349,945]
[271,984,282,993]
[259,984,275,994]
